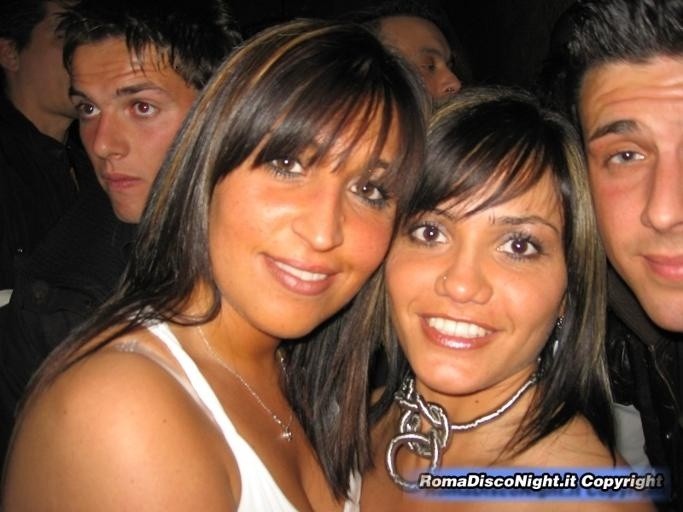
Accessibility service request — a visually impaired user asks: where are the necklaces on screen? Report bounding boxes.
[386,373,549,493]
[188,314,295,442]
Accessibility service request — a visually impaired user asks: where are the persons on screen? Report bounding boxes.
[559,2,683,507]
[349,88,656,509]
[369,13,464,117]
[0,0,77,381]
[2,20,425,510]
[60,3,242,456]
